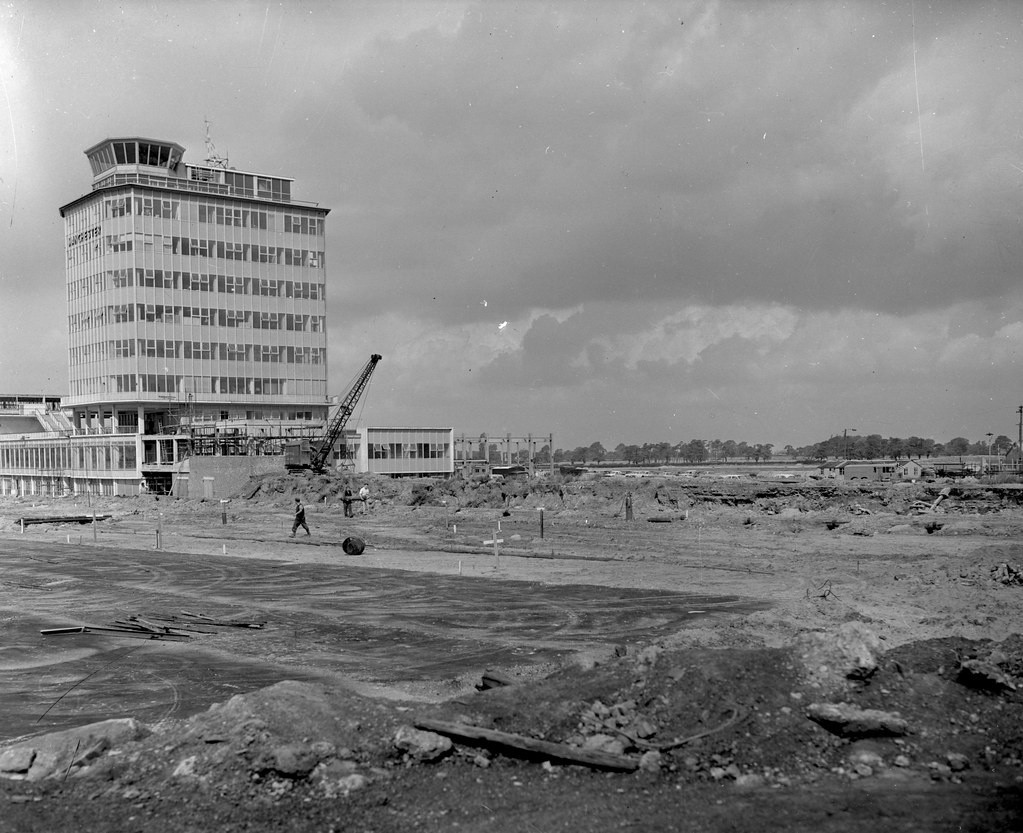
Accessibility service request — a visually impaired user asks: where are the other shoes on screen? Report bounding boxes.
[363,513,367,515]
[350,515,353,518]
[345,514,349,517]
[304,533,311,536]
[289,534,296,538]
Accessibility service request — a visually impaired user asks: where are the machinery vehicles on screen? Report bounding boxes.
[283,354,382,476]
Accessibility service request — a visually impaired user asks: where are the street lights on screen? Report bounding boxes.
[843,428,857,459]
[986,432,994,479]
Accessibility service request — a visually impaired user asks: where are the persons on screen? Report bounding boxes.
[1018,457,1022,464]
[342,486,356,518]
[44,400,49,415]
[962,463,966,468]
[358,483,371,515]
[289,497,312,538]
[999,458,1003,465]
[140,482,148,494]
[256,427,267,454]
